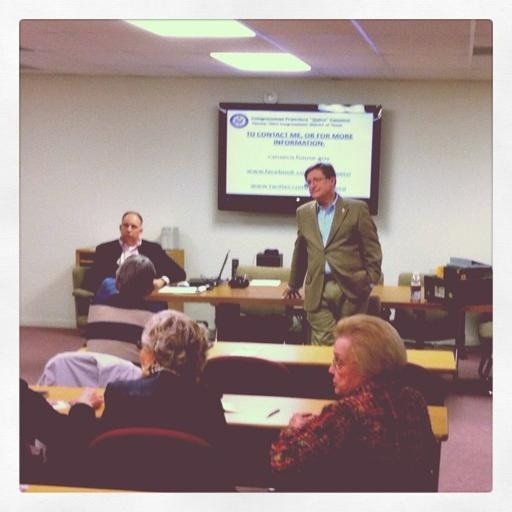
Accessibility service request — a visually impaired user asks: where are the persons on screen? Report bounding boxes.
[266,312,438,492]
[92,308,239,493]
[282,162,382,349]
[80,210,186,310]
[84,254,159,368]
[20,374,101,486]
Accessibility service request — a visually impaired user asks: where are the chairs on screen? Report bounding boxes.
[82,428,221,492]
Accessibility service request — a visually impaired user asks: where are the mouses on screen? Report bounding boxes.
[177,281,189,287]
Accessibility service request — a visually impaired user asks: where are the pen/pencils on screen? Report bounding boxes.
[267,409,280,418]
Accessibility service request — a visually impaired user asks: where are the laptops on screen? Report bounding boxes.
[190,249,230,285]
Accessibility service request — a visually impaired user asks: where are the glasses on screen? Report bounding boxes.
[136,339,144,349]
[331,358,346,369]
[306,176,325,187]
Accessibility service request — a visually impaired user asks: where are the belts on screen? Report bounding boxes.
[324,272,336,282]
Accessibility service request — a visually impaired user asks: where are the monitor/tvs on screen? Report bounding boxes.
[218,103,381,214]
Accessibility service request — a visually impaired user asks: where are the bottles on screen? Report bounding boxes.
[409,270,423,304]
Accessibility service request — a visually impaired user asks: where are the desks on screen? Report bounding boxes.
[29,248,492,444]
